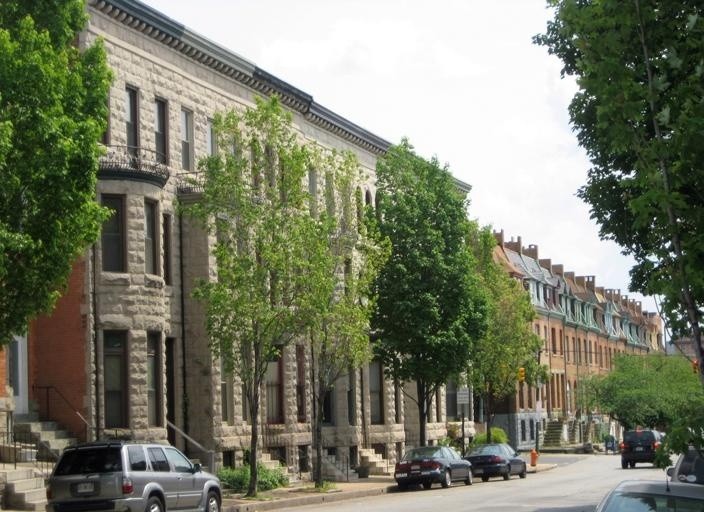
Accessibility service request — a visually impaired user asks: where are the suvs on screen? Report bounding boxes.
[620,429,662,469]
[44,440,224,511]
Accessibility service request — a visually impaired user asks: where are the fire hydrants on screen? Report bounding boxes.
[531,448,539,466]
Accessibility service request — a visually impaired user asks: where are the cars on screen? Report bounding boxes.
[394,445,473,490]
[461,443,526,482]
